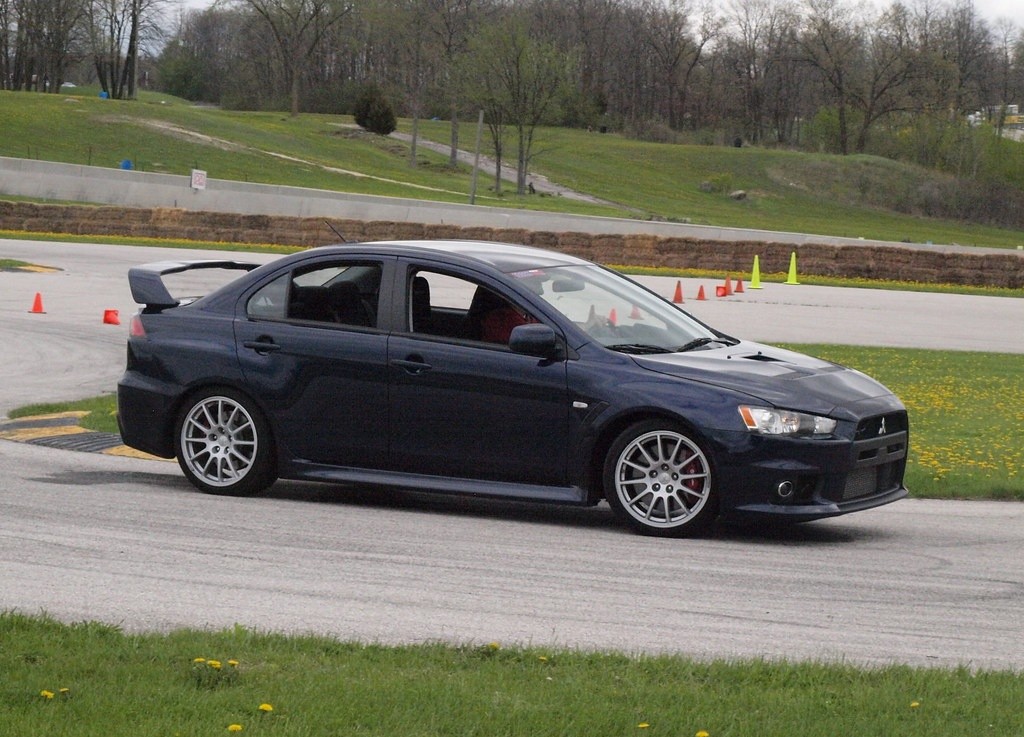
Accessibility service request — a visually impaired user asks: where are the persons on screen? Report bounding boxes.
[529,182,535,194]
[479,282,544,346]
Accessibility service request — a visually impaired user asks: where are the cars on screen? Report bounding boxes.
[115,238,910,539]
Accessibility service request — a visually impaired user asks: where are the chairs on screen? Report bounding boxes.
[412,277,497,338]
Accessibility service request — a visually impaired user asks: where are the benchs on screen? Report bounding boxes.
[288,280,377,327]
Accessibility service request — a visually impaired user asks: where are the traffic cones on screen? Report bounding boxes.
[28,291,47,314]
[628,304,644,319]
[672,280,685,303]
[723,273,735,296]
[716,287,726,297]
[746,255,764,289]
[696,286,707,300]
[784,252,801,285]
[104,310,120,326]
[736,277,744,292]
[607,309,618,324]
